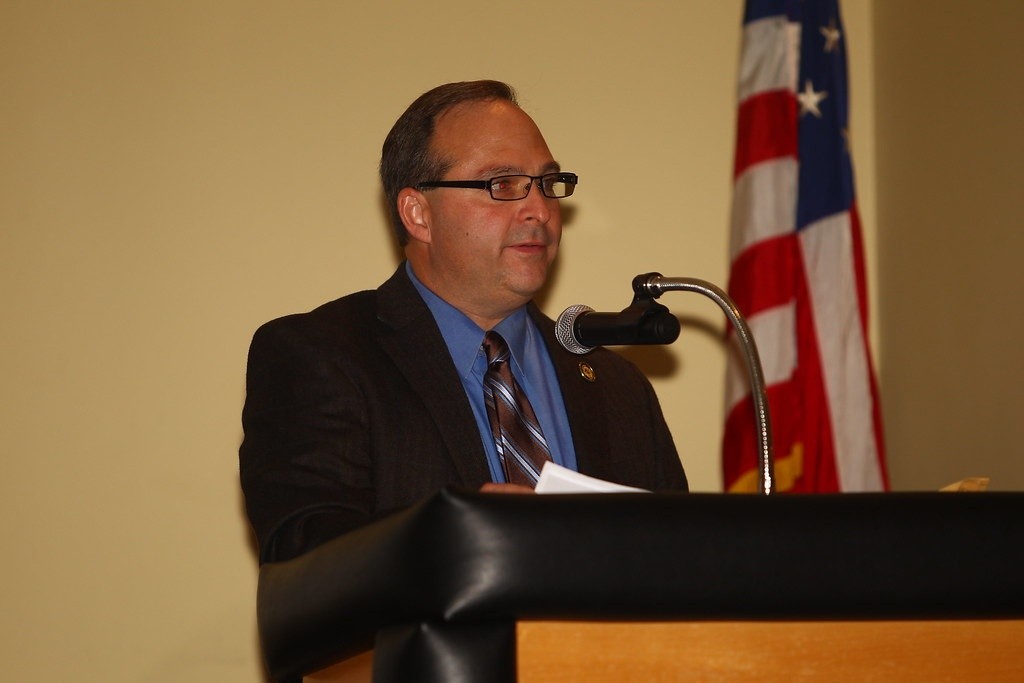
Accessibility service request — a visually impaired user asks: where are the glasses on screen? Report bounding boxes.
[416,172,578,200]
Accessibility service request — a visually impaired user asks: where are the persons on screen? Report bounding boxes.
[239,80,691,570]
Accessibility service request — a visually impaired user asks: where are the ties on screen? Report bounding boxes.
[480,330,552,492]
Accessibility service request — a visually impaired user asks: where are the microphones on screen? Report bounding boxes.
[554,301,682,357]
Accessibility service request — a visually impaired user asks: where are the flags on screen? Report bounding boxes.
[717,0,895,494]
